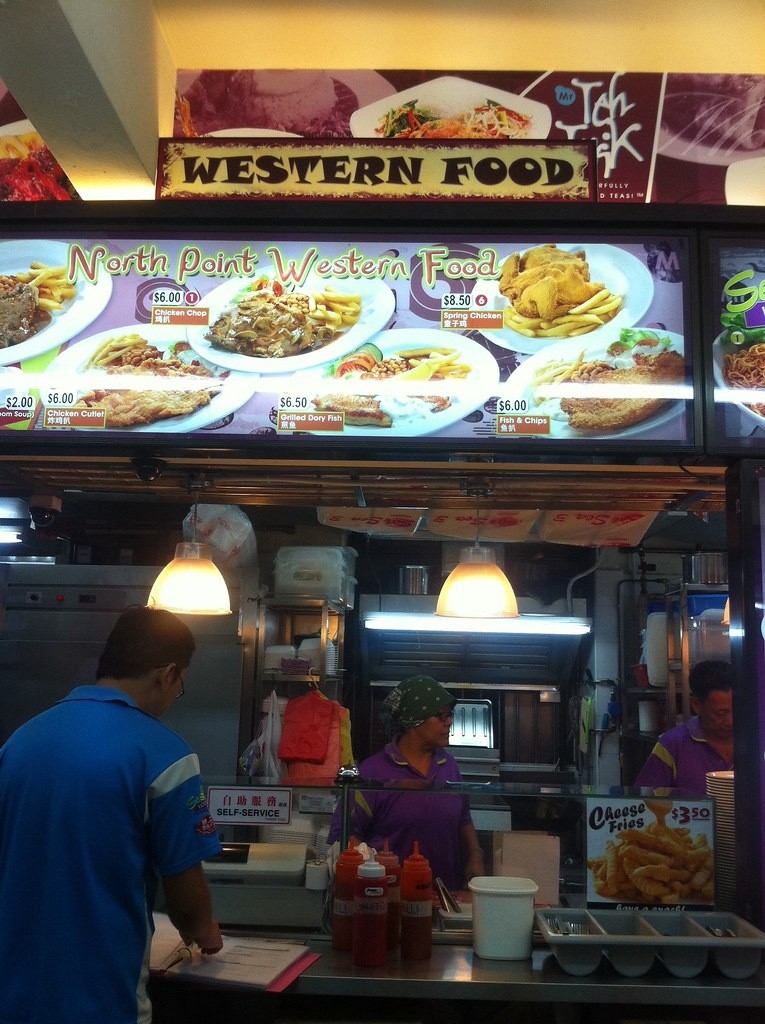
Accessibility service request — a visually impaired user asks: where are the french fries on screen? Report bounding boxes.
[501,288,623,338]
[529,348,585,405]
[396,346,471,374]
[84,333,148,369]
[587,822,715,903]
[14,260,77,310]
[309,283,362,327]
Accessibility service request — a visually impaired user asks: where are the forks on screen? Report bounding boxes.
[547,915,590,934]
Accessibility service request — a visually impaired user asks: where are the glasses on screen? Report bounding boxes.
[174,667,185,699]
[432,711,454,721]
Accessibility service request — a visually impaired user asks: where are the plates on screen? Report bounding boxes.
[706,771,735,914]
[0,241,685,435]
[174,69,551,141]
[714,327,765,425]
[438,904,473,921]
[0,119,80,201]
[260,819,330,854]
[264,638,339,676]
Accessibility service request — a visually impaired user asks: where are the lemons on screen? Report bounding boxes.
[390,362,432,385]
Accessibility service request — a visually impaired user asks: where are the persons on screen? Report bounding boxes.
[0,603,223,1024]
[327,674,486,890]
[630,660,734,799]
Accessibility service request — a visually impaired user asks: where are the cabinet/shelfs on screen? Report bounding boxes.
[248,596,345,837]
[666,580,729,729]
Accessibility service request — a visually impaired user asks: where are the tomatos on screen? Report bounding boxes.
[336,352,376,380]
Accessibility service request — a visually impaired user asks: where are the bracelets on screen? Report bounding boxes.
[467,847,484,855]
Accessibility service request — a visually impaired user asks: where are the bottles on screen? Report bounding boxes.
[332,836,434,965]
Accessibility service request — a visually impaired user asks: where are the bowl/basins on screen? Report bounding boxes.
[280,657,314,676]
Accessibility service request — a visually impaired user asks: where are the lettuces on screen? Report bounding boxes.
[607,328,672,357]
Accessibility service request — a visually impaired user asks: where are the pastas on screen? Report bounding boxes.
[722,340,765,420]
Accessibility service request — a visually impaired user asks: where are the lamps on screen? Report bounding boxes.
[144,488,233,618]
[435,478,521,619]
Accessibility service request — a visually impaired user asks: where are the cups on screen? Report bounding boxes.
[469,877,539,961]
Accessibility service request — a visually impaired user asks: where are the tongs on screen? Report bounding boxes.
[435,878,463,913]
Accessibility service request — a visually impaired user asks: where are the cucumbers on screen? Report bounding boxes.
[356,344,383,361]
